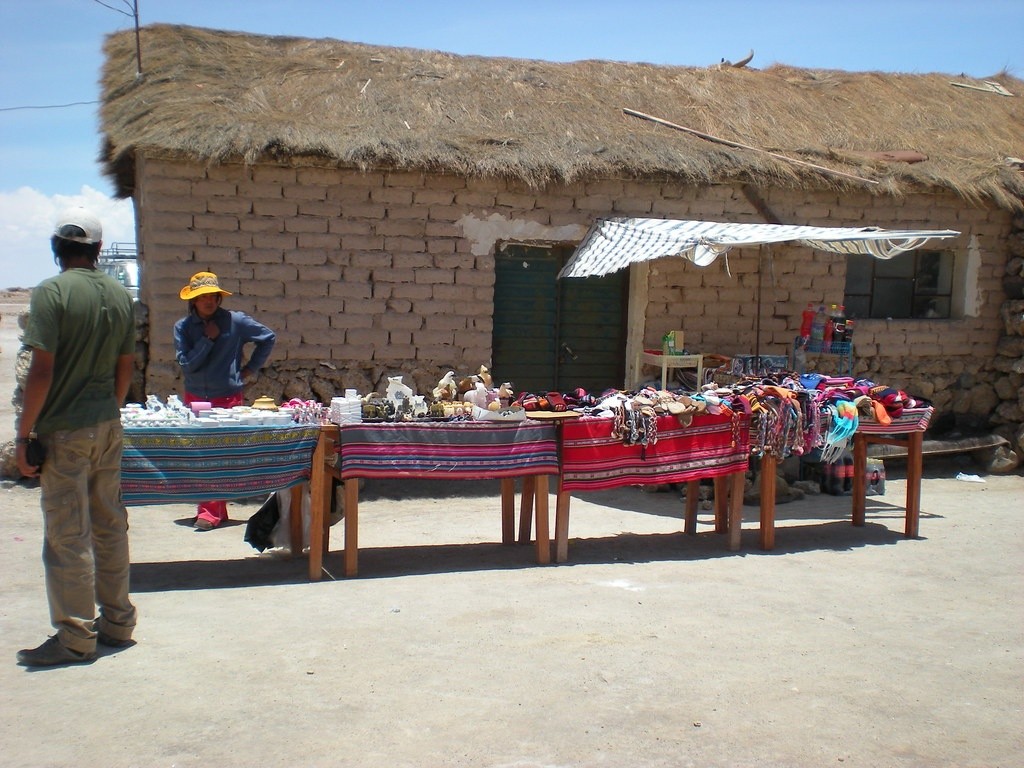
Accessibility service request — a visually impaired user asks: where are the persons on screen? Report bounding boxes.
[173,271,274,530]
[8,215,137,666]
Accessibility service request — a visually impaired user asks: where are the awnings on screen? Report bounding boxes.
[554,217,960,278]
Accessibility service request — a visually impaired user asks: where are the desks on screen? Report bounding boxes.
[709,384,936,550]
[116,407,339,583]
[319,406,559,580]
[518,390,752,564]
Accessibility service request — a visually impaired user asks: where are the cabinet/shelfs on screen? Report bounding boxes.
[634,345,704,394]
[791,335,854,380]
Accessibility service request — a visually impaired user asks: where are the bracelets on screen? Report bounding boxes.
[13,438,30,443]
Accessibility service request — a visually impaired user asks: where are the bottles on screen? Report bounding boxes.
[831,305,846,354]
[662,330,675,355]
[842,319,855,354]
[823,320,835,354]
[799,450,886,497]
[811,306,825,351]
[801,304,817,339]
[827,305,837,321]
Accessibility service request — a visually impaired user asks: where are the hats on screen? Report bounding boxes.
[51,207,103,245]
[179,271,232,300]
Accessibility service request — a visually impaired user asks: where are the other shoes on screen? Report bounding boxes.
[16,635,98,667]
[95,620,132,648]
[194,517,214,531]
